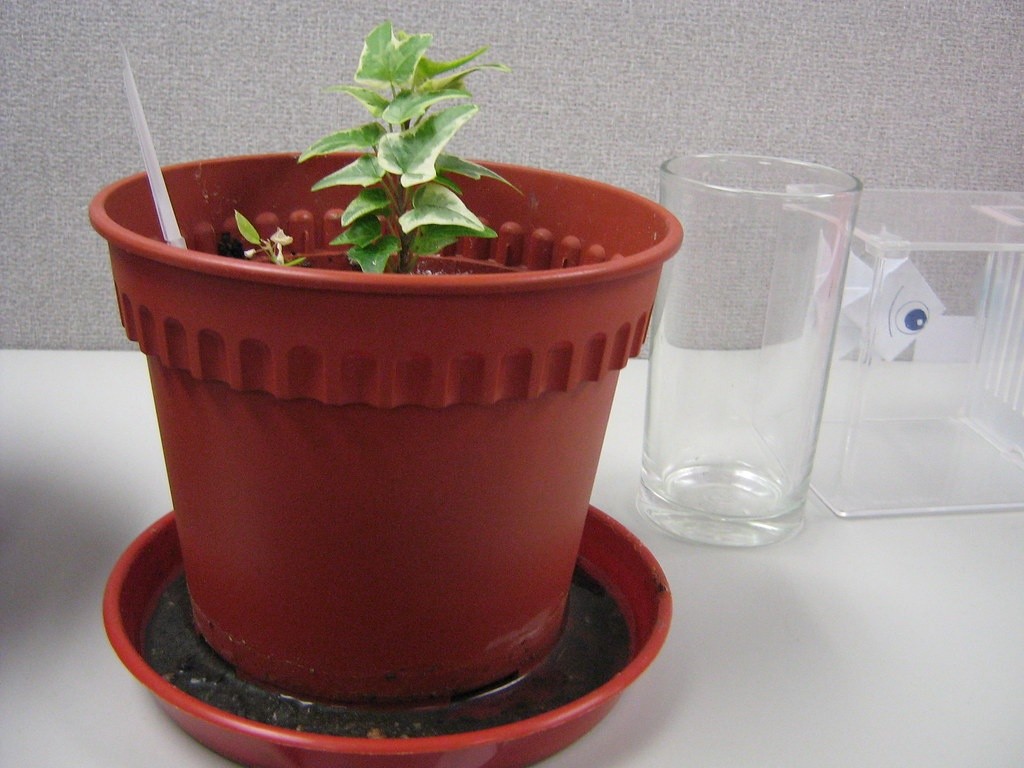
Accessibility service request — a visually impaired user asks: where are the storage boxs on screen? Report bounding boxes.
[746,188,1024,523]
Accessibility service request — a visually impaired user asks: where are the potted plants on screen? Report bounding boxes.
[86,19,686,710]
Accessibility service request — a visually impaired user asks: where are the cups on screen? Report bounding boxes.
[638,153,864,547]
